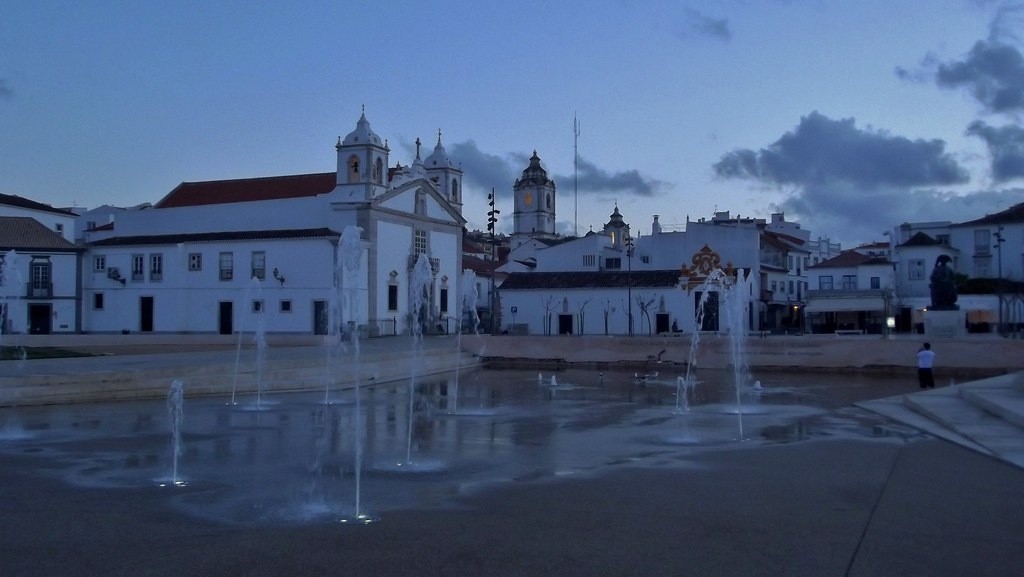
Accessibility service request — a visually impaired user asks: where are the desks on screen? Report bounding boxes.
[716,330,745,335]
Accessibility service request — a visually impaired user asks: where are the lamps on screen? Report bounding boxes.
[273,268,285,286]
[112,271,127,287]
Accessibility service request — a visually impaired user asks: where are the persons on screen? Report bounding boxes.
[929,253,958,310]
[915,343,936,390]
[672,321,683,337]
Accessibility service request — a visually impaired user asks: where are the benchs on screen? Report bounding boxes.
[747,331,770,336]
[834,329,863,334]
[660,332,686,337]
[687,331,715,335]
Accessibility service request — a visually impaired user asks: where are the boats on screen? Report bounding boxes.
[635,350,696,387]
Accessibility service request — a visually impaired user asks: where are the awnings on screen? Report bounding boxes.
[967,310,996,323]
[913,310,924,324]
[804,298,885,311]
